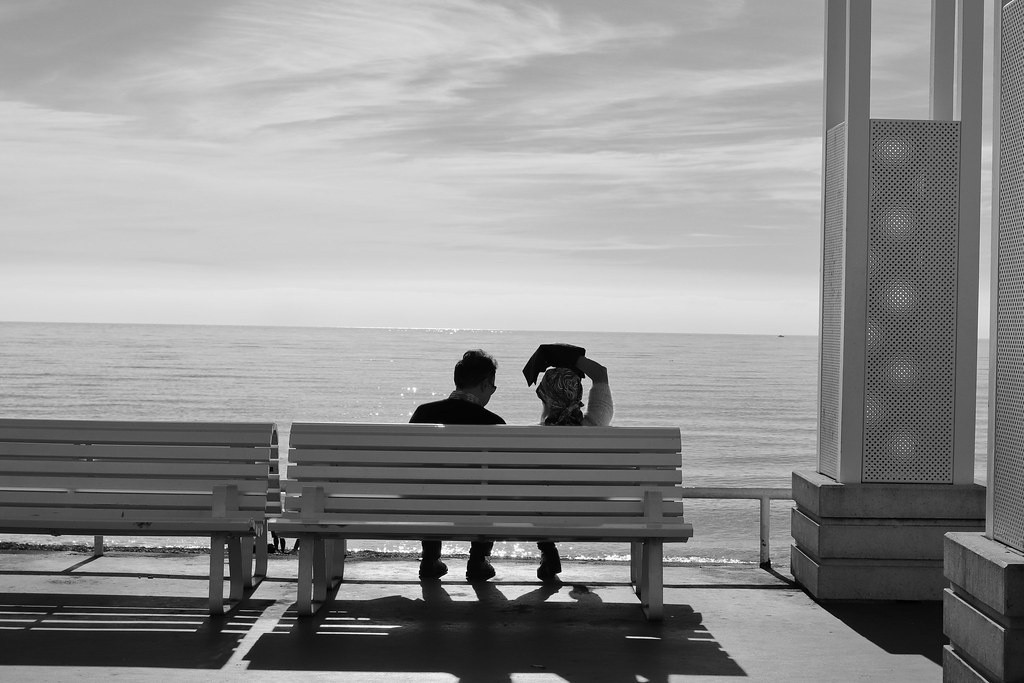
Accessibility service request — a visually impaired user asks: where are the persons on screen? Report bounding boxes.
[526,343,614,577]
[408,348,508,584]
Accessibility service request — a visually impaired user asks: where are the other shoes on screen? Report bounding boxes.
[466,560,496,581]
[419,559,448,579]
[537,554,561,579]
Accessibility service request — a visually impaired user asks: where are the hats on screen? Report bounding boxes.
[536,368,584,425]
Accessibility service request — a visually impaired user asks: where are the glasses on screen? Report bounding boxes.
[490,385,496,395]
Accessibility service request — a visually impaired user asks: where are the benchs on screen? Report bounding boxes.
[0,417,283,622]
[267,421,694,626]
